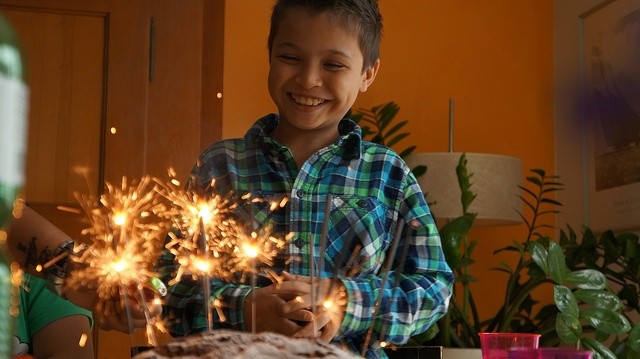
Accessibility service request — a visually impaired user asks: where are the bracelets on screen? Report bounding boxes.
[51,239,75,279]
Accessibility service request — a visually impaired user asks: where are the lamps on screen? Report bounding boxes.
[400,98,525,224]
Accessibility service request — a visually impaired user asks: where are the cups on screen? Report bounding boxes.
[477,331,543,359]
[536,347,592,359]
[505,346,557,359]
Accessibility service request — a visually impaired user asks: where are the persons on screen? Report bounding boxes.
[0,197,165,358]
[157,0,456,358]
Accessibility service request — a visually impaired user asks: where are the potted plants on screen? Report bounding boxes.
[347,100,640,359]
